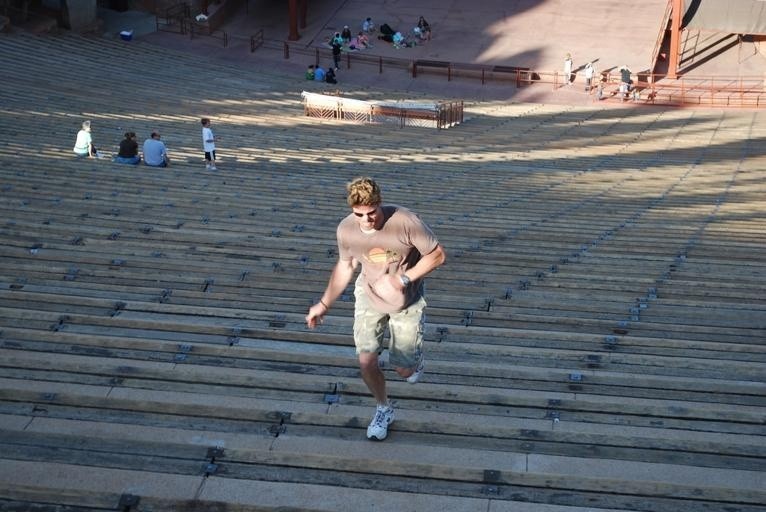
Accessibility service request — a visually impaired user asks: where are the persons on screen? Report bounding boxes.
[306,177,446,440]
[619,65,633,102]
[305,65,337,84]
[417,16,431,43]
[201,118,221,172]
[329,17,380,70]
[115,131,140,164]
[73,120,102,158]
[584,62,608,101]
[564,52,574,85]
[143,130,169,167]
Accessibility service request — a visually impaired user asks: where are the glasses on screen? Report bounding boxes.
[353,208,377,217]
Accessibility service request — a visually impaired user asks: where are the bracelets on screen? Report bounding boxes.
[318,299,329,311]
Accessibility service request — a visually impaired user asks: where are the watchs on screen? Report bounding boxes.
[400,274,411,287]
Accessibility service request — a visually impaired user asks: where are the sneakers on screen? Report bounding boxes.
[367,405,395,440]
[406,363,424,383]
